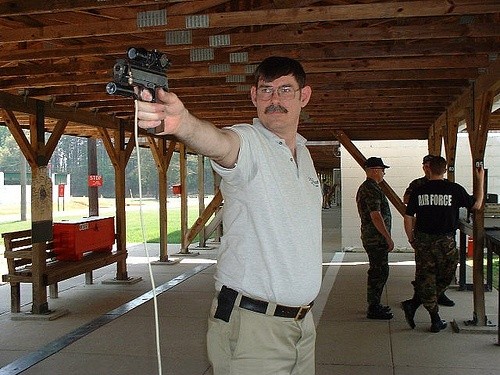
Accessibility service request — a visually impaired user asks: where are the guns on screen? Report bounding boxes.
[105,47,171,135]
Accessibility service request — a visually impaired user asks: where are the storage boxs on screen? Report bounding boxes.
[54,215,116,261]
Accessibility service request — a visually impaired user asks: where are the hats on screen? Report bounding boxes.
[422,154,434,163]
[364,157,390,169]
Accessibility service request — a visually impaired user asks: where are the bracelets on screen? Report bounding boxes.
[408,238,415,244]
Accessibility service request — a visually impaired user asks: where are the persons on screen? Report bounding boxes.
[403,154,457,306]
[135,56,324,375]
[400,153,484,332]
[320,179,339,210]
[356,157,394,320]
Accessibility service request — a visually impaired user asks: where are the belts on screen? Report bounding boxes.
[239,296,314,321]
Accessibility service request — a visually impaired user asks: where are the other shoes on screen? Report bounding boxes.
[379,306,391,312]
[367,310,394,320]
[437,292,455,307]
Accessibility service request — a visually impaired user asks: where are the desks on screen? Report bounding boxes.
[457,216,500,346]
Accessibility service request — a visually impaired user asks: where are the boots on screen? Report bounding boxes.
[430,314,447,333]
[401,298,419,330]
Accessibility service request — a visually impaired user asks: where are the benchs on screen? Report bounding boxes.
[1,228,129,312]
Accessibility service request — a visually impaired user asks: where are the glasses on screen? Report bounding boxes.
[257,86,304,100]
